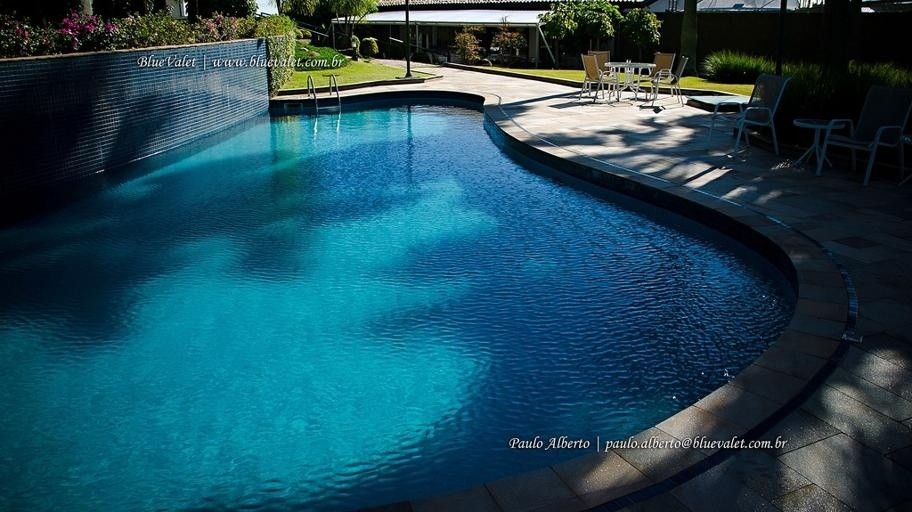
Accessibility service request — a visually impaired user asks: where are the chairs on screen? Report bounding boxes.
[704,75,912,178]
[579,51,689,107]
